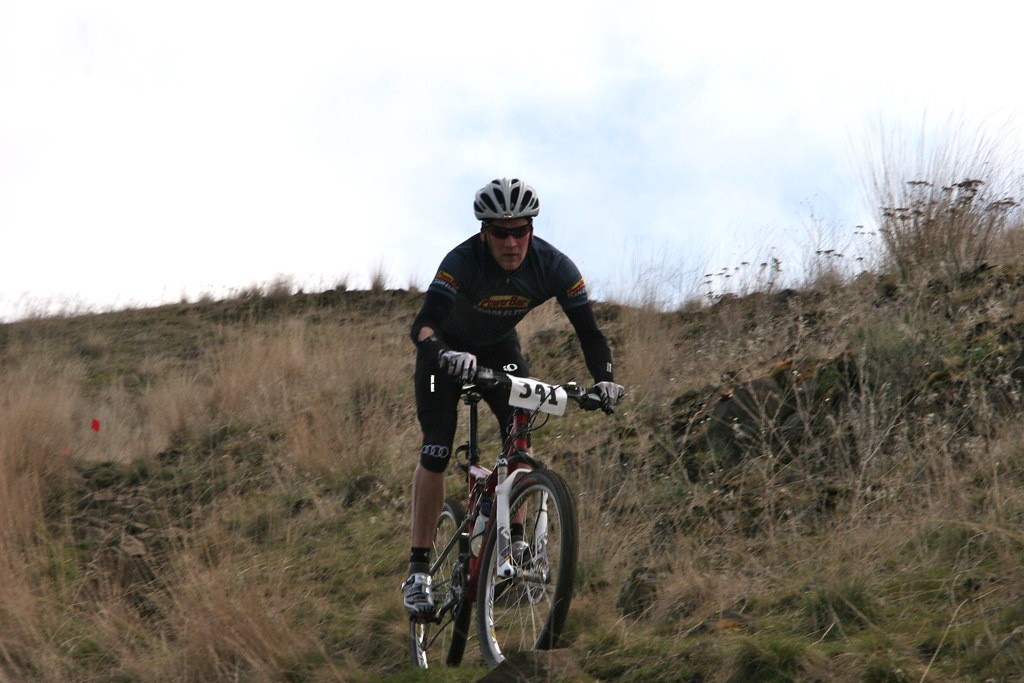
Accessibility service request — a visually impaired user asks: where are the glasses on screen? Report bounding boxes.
[482,220,534,238]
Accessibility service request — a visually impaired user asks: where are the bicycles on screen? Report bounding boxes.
[408,356,600,670]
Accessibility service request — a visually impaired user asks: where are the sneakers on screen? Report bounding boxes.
[400,575,436,614]
[511,542,545,576]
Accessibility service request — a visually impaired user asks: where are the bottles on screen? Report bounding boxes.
[471,493,495,558]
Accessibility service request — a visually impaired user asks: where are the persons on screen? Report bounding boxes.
[403,177,624,614]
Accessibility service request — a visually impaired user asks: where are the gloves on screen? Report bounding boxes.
[439,351,478,381]
[594,380,627,411]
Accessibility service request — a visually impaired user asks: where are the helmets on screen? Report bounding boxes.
[474,178,540,218]
